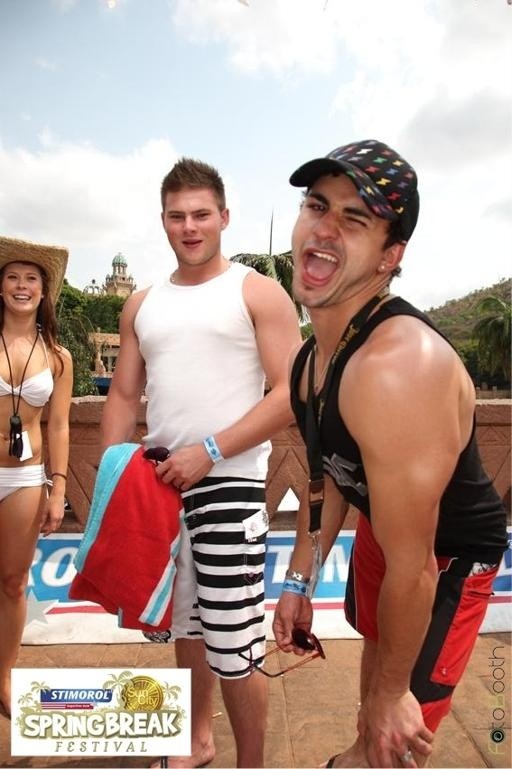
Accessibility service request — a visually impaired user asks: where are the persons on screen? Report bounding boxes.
[272,139,509,769]
[99,157,303,769]
[0,235,73,720]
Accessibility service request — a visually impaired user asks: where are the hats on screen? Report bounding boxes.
[288,138,419,242]
[1,237,68,309]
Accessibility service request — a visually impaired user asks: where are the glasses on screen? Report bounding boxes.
[145,446,171,465]
[9,416,23,458]
[239,628,326,677]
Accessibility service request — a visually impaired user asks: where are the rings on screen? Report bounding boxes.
[401,751,412,763]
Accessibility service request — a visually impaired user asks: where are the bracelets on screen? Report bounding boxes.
[285,569,308,584]
[282,579,306,595]
[203,436,225,465]
[51,473,67,480]
